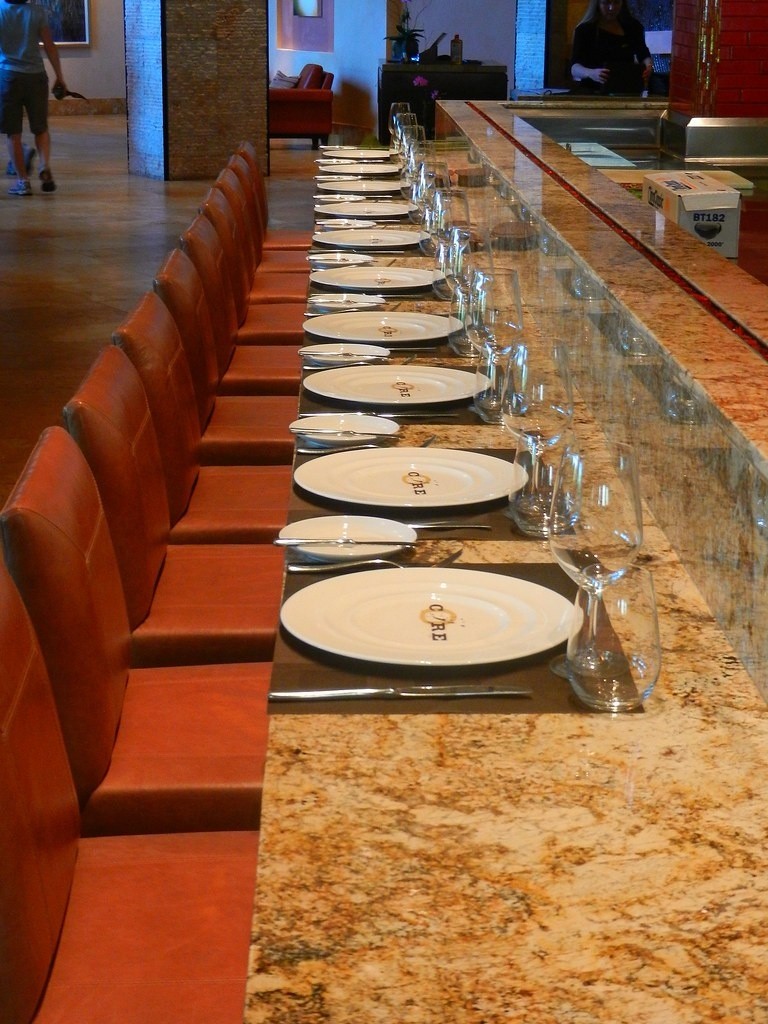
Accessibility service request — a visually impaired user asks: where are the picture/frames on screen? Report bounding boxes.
[26,0,89,47]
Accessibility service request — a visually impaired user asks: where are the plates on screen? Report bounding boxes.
[278,145,585,668]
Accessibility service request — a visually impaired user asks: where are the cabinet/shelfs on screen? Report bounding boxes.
[379,70,507,149]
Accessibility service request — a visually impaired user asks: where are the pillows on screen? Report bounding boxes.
[269,72,301,92]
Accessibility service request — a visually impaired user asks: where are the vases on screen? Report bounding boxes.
[402,41,419,63]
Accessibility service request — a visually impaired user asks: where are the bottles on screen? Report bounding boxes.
[450,34,463,65]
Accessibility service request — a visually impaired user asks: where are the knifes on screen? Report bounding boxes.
[385,346,437,351]
[289,428,406,439]
[315,159,383,163]
[313,175,387,179]
[307,249,404,254]
[315,218,401,223]
[375,293,423,299]
[265,686,534,702]
[312,194,393,199]
[404,520,492,529]
[272,537,423,547]
[298,350,388,360]
[298,410,459,419]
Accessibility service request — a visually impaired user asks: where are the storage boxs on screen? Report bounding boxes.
[641,170,739,261]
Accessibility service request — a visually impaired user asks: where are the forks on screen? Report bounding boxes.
[286,549,463,572]
[297,435,437,454]
[303,302,401,317]
[302,353,417,370]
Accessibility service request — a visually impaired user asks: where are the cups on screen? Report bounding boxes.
[384,96,663,715]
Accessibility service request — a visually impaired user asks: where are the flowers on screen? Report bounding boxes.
[382,0,426,48]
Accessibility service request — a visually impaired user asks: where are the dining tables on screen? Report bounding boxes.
[243,143,767,1024]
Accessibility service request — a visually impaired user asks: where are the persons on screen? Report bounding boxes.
[570,0,654,94]
[0,0,68,195]
[6,143,36,174]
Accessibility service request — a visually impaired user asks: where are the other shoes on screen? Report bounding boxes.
[8,179,33,195]
[38,164,55,193]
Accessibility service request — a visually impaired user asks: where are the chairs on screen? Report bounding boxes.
[0,137,267,1023]
[268,64,335,148]
